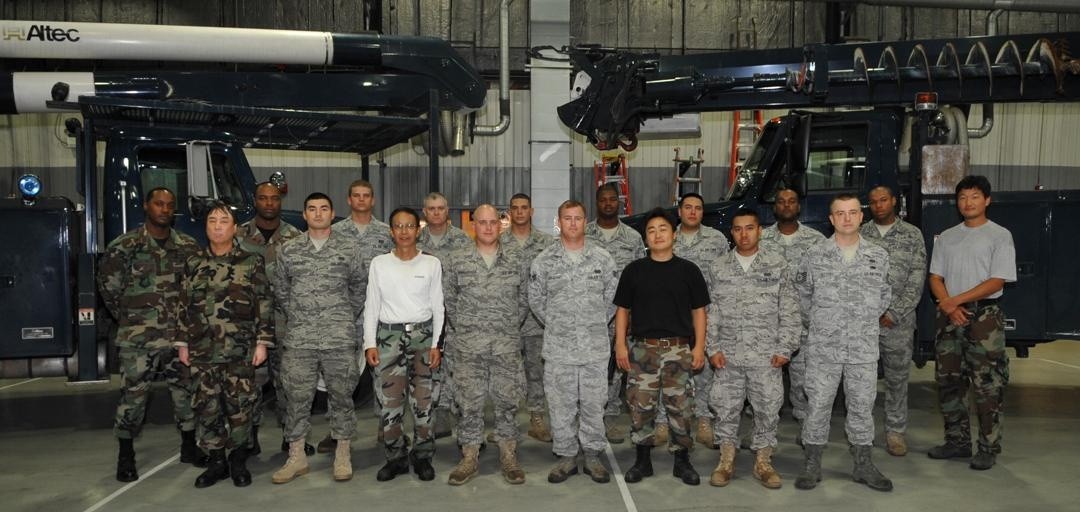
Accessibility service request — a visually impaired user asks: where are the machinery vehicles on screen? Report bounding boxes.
[520,33,1078,417]
[1,21,492,416]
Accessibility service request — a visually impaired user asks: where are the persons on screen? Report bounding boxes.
[94,184,929,491]
[927,175,1018,471]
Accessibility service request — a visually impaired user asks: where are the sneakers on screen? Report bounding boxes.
[583,451,610,482]
[884,430,908,457]
[548,456,579,482]
[740,424,754,449]
[777,423,785,443]
[969,450,998,471]
[925,439,973,460]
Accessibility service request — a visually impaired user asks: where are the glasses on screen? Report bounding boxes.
[391,221,420,232]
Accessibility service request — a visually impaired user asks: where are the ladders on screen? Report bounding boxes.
[671,147,705,209]
[594,151,632,219]
[729,109,763,189]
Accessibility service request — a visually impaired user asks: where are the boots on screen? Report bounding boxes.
[651,421,668,448]
[228,445,252,488]
[282,425,315,456]
[528,414,552,442]
[334,440,353,479]
[179,428,210,469]
[694,416,717,450]
[794,419,805,444]
[496,440,525,482]
[708,442,737,487]
[272,438,308,482]
[448,445,480,485]
[752,447,783,490]
[434,409,452,438]
[625,443,654,484]
[604,415,621,444]
[849,442,895,492]
[793,443,826,490]
[192,445,230,489]
[671,448,700,485]
[227,424,262,462]
[487,412,520,442]
[114,435,138,484]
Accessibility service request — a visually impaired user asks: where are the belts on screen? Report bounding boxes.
[378,314,434,332]
[956,294,1006,307]
[633,335,690,349]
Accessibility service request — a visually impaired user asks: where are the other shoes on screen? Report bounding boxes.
[317,434,337,452]
[377,455,409,481]
[378,428,411,445]
[414,459,435,481]
